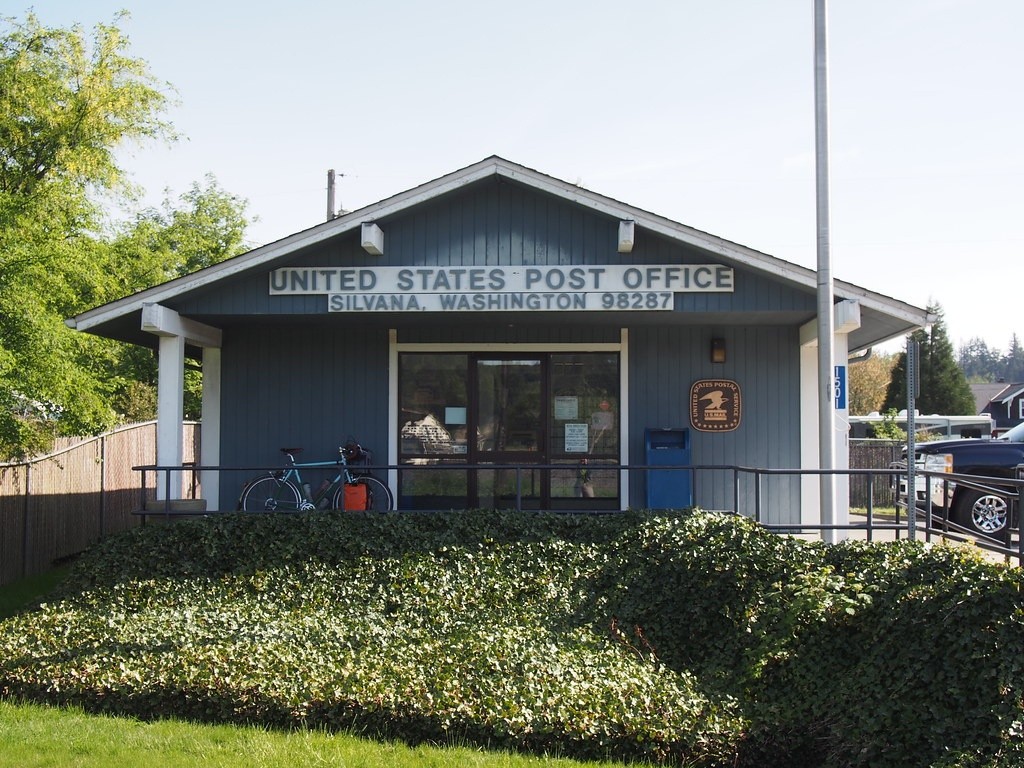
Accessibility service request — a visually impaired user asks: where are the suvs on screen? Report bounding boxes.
[888,417,1024,544]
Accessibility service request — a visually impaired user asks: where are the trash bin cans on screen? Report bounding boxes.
[643,426,694,510]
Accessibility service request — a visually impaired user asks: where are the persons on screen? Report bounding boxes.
[576,459,594,497]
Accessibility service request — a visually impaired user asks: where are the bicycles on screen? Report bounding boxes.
[226,438,398,517]
